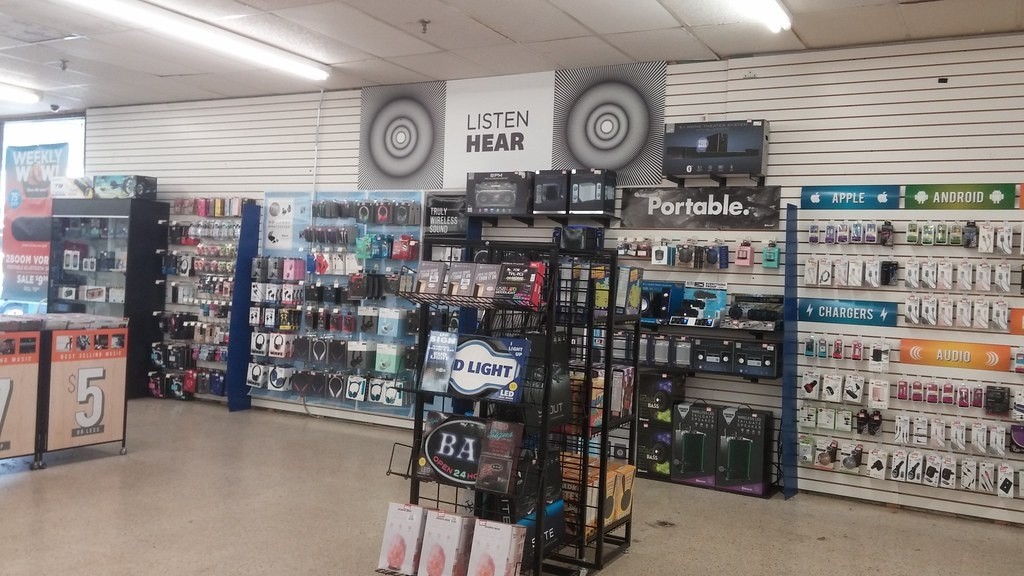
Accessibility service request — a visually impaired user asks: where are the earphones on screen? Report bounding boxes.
[251,256,306,330]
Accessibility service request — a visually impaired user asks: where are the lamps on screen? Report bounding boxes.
[735,0,794,34]
[0,82,40,107]
[51,0,332,83]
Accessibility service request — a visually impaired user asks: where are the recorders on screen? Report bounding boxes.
[475,182,517,208]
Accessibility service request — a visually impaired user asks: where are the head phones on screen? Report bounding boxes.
[294,311,397,406]
[448,317,459,333]
[358,206,369,222]
[377,206,388,221]
[255,335,264,349]
[269,368,287,388]
[252,365,261,381]
[178,259,188,273]
[397,206,407,222]
[274,335,283,350]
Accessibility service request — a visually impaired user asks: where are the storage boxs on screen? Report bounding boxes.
[145,311,225,400]
[466,171,535,214]
[410,260,545,311]
[533,169,571,215]
[377,501,440,576]
[662,117,770,178]
[636,244,783,496]
[483,258,644,570]
[414,410,525,496]
[569,169,618,215]
[799,220,1017,499]
[56,248,108,302]
[467,518,528,576]
[49,175,157,199]
[418,330,532,403]
[245,199,421,407]
[417,510,479,576]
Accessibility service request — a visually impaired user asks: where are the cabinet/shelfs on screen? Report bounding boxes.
[47,198,170,401]
[383,233,643,576]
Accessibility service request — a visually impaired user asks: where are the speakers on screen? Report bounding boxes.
[694,348,707,363]
[762,355,775,368]
[719,431,752,483]
[674,428,706,475]
[735,353,747,367]
[595,183,602,201]
[720,351,731,366]
[571,183,578,204]
